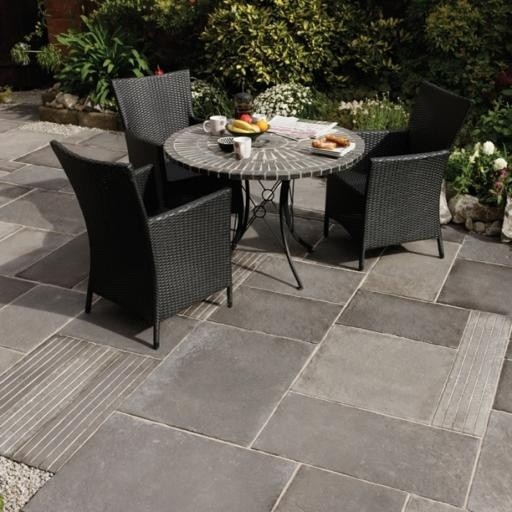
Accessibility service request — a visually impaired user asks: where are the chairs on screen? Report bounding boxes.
[110,68,244,214]
[51,139,233,348]
[323,79,476,271]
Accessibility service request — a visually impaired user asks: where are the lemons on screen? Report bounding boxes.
[257,119,267,131]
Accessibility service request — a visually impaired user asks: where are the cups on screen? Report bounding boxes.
[202,114,227,136]
[233,136,253,161]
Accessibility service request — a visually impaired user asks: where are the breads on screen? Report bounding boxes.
[312,134,350,150]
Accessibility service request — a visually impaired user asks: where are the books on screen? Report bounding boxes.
[267,115,340,143]
[305,141,356,158]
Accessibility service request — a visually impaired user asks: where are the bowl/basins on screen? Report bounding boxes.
[218,137,234,152]
[226,118,271,142]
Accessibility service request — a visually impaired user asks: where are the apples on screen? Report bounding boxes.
[240,114,252,123]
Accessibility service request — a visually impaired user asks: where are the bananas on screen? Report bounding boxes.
[232,120,256,133]
